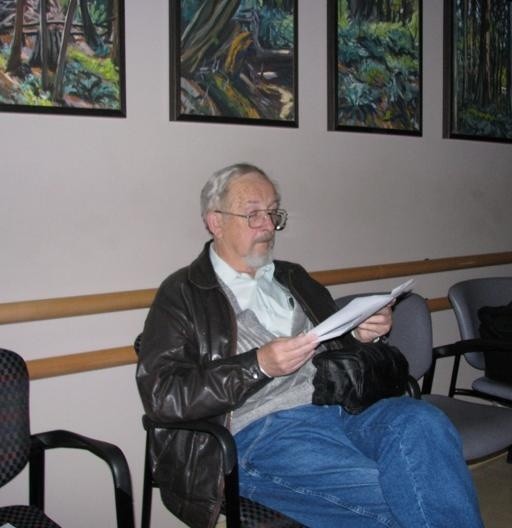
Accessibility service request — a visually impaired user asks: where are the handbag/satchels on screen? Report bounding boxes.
[311,345,409,416]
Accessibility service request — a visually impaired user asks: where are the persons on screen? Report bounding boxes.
[135,163,484,528]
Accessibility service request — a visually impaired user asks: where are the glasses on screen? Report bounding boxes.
[214,209,288,230]
